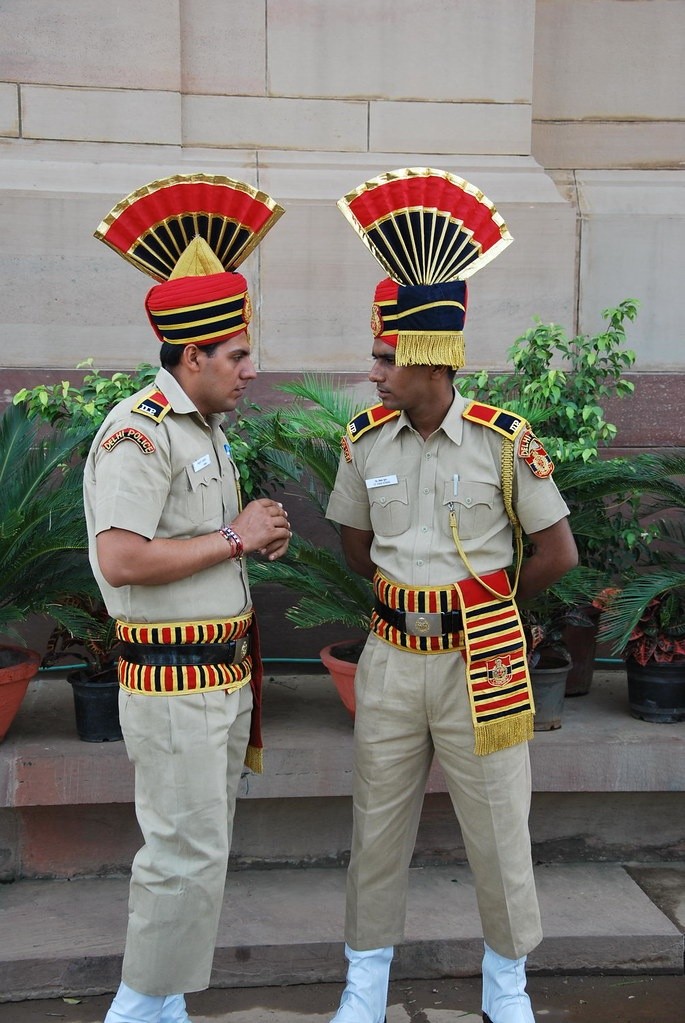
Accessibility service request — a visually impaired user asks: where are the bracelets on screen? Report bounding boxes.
[219,527,244,561]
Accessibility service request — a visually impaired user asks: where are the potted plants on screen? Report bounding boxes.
[1,298,684,743]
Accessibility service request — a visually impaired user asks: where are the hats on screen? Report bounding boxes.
[340,167,511,369]
[99,169,282,343]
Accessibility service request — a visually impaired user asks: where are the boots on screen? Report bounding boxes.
[326,944,393,1023]
[483,946,536,1023]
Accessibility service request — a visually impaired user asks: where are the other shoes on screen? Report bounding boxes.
[103,981,193,1023]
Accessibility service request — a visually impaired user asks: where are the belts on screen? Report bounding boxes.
[120,637,251,667]
[373,600,462,638]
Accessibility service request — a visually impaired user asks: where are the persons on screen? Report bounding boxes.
[325,278,579,1023]
[83,273,291,1022]
